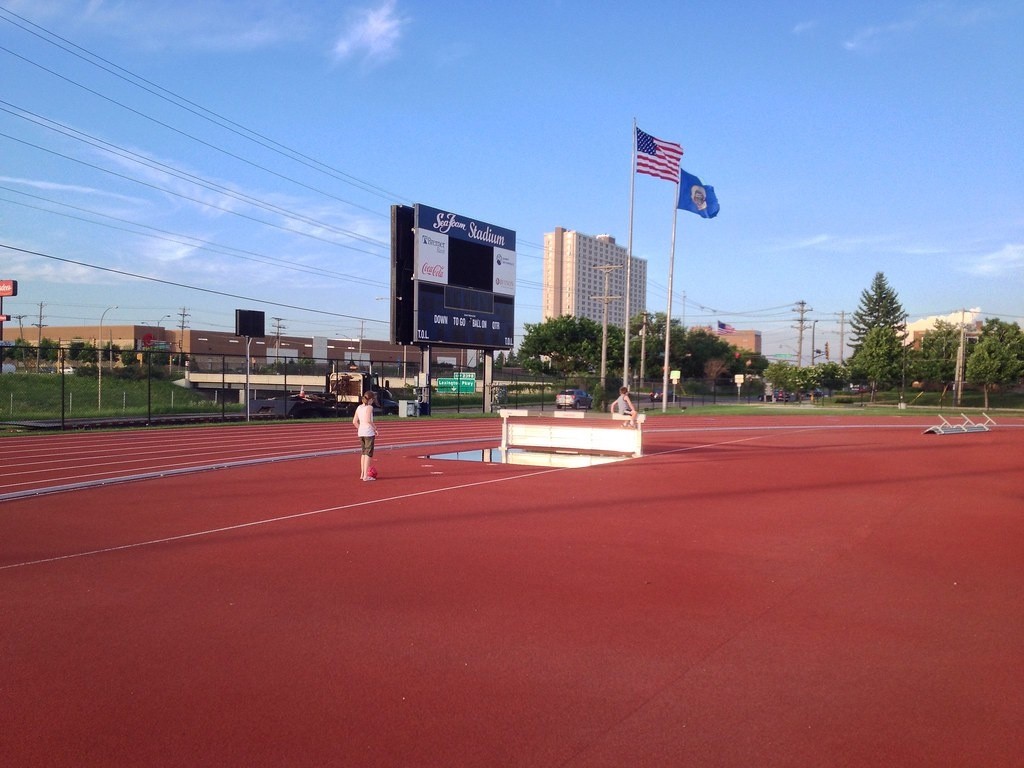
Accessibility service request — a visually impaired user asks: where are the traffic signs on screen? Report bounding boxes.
[437,372,476,394]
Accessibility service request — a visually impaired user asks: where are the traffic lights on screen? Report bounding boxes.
[825,341,830,360]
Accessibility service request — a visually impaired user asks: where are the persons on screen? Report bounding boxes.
[610,386,638,429]
[352,391,378,481]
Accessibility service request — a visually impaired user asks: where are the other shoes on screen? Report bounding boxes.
[621,424,627,429]
[363,476,376,481]
[628,424,636,430]
[360,477,363,479]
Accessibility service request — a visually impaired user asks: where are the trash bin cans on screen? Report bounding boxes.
[419,402,429,416]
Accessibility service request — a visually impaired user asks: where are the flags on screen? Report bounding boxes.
[718,322,736,336]
[676,169,720,219]
[636,127,683,183]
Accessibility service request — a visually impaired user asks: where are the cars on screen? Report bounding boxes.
[650,387,678,403]
[58,366,78,375]
[555,388,595,410]
[851,387,859,394]
[807,388,823,398]
[758,391,790,402]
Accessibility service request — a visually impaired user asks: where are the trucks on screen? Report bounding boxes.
[240,370,399,422]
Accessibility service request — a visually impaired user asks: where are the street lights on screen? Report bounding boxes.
[141,314,171,368]
[812,320,819,365]
[98,306,119,413]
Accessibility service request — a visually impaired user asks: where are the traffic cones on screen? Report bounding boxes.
[298,385,305,398]
